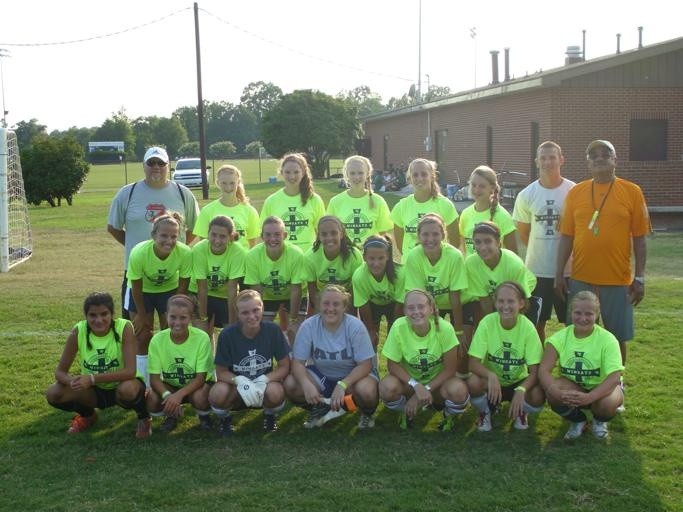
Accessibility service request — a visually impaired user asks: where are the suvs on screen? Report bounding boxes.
[169,158,212,189]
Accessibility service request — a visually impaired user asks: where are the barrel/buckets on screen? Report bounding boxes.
[447,183,456,199]
[269,176,276,183]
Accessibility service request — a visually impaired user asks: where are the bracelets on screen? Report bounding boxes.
[88,374,95,386]
[633,276,645,284]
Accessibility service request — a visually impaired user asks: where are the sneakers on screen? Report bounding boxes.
[67,410,97,434]
[513,409,529,430]
[136,417,153,440]
[220,415,232,439]
[397,412,413,431]
[592,414,608,440]
[564,419,590,439]
[199,414,212,432]
[159,416,177,433]
[358,412,375,432]
[477,411,492,432]
[303,407,332,427]
[262,411,278,434]
[438,411,455,431]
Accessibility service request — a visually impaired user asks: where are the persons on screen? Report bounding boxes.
[554,139,653,413]
[537,290,625,439]
[46,291,152,439]
[106,147,199,319]
[512,141,577,346]
[284,153,543,434]
[123,154,329,435]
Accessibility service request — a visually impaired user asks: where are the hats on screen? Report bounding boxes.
[585,139,615,156]
[144,146,169,164]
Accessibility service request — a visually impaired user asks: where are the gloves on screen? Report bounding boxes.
[232,376,259,407]
[253,374,270,406]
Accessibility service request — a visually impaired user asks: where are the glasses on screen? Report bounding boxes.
[589,151,610,160]
[147,160,167,167]
